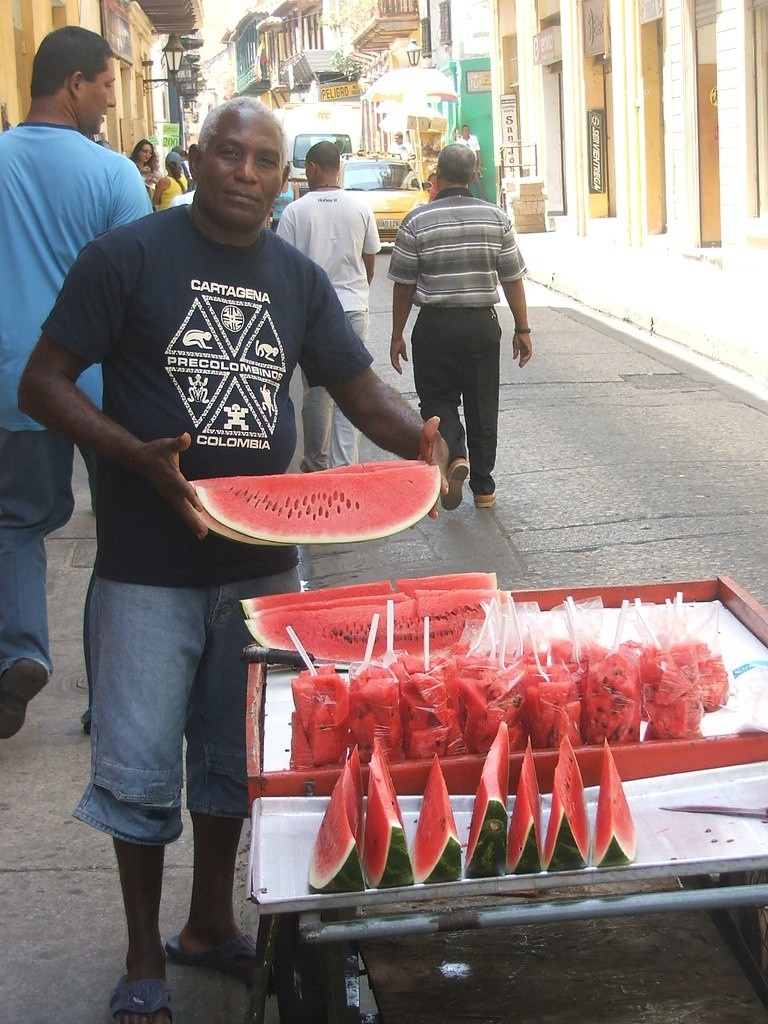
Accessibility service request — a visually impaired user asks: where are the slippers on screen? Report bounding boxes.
[164,933,257,981]
[110,974,175,1024]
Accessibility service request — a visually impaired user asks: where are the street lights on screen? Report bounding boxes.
[162,34,187,148]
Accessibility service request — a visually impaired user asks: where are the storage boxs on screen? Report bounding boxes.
[504,179,549,234]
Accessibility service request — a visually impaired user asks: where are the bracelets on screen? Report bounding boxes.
[514,327,532,334]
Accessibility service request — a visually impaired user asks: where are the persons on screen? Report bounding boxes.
[16,95,452,1024]
[270,163,300,233]
[455,125,489,202]
[0,26,155,739]
[277,138,381,474]
[386,143,533,511]
[128,139,196,212]
[390,132,416,162]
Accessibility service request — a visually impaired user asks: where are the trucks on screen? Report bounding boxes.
[280,102,362,202]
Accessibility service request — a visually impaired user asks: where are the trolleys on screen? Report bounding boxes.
[244,570,768,1024]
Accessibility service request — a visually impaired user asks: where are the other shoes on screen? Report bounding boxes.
[441,458,469,511]
[472,489,495,506]
[0,657,50,739]
[298,458,314,473]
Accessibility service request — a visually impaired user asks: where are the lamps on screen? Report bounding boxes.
[405,39,423,67]
[143,32,186,96]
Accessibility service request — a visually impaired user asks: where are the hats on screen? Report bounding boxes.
[165,152,181,166]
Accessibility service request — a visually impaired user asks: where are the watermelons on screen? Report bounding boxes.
[240,572,511,671]
[289,636,729,769]
[308,722,637,893]
[186,461,441,547]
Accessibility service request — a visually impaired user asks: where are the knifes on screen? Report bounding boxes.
[659,803,768,817]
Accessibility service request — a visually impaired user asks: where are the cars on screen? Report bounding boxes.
[338,156,432,242]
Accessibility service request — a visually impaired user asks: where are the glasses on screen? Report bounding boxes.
[141,149,153,154]
